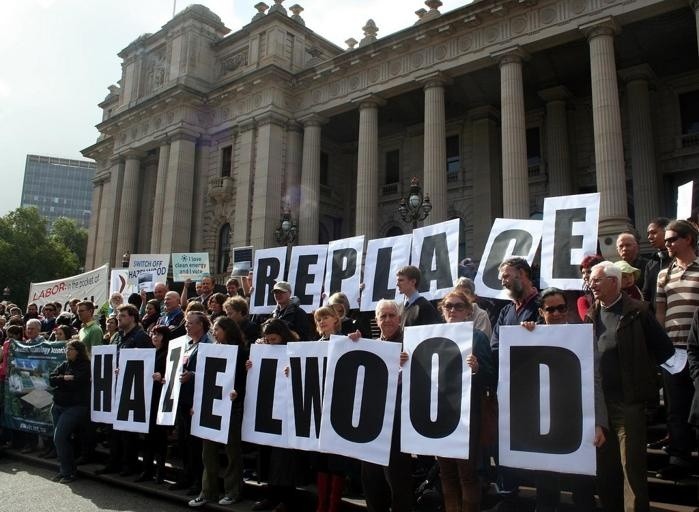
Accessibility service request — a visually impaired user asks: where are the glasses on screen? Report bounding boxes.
[588,277,612,288]
[8,335,14,338]
[66,348,77,351]
[70,304,77,307]
[541,303,569,314]
[44,307,54,312]
[77,309,89,313]
[442,302,467,312]
[664,235,681,244]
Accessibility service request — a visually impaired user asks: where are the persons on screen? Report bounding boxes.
[0,217,698,511]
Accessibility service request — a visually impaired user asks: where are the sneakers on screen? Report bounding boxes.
[57,473,79,483]
[217,493,243,506]
[187,489,219,508]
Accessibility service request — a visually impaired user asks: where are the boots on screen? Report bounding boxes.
[328,475,344,511]
[314,470,328,511]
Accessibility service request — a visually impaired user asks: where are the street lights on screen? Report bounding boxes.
[121,252,128,268]
[1,285,10,301]
[398,175,432,231]
[273,207,298,249]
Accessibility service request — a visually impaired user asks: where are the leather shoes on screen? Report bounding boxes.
[644,429,694,481]
[250,490,282,512]
[271,495,301,512]
[93,461,202,496]
[1,440,92,465]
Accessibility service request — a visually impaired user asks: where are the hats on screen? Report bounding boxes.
[270,280,292,294]
[613,260,641,281]
